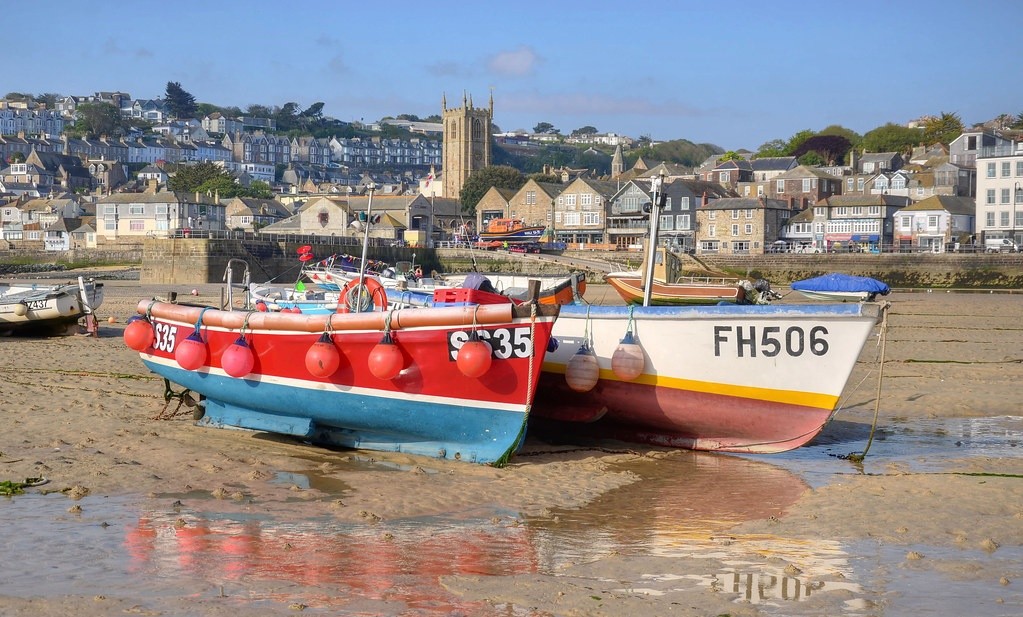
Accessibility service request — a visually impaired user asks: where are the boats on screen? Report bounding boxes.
[242,273,417,315]
[246,167,893,456]
[601,245,785,306]
[790,272,891,302]
[478,216,547,246]
[0,275,104,337]
[134,258,564,468]
[300,250,589,306]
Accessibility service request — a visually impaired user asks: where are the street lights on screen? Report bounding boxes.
[1012,181,1022,252]
[759,193,768,253]
[879,186,888,253]
[550,197,556,248]
[598,198,608,245]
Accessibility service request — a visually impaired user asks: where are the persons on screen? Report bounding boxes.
[503,240,509,248]
[306,290,315,300]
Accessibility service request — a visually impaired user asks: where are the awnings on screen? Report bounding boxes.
[825,232,878,242]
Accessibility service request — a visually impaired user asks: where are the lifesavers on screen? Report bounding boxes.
[337,277,387,313]
[416,269,422,275]
[663,239,669,245]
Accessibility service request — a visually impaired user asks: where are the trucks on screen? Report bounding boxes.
[985,238,1022,254]
